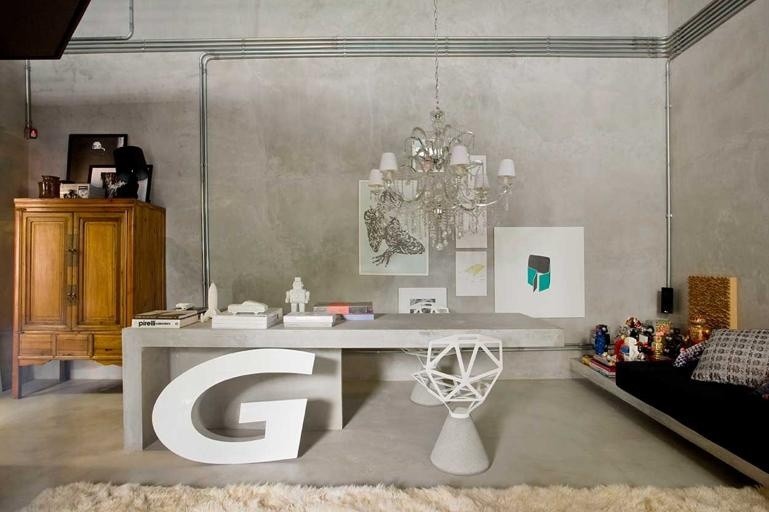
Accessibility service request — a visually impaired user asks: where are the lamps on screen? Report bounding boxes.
[364,7,517,252]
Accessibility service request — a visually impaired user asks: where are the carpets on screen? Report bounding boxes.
[19,480,764,512]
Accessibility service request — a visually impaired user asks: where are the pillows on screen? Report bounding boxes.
[695,323,768,388]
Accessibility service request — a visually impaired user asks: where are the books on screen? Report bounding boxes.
[313,302,375,322]
[131,309,199,329]
[283,311,337,328]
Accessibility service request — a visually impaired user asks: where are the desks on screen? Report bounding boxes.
[113,308,567,456]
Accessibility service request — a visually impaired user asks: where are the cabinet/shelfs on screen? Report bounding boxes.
[13,195,166,399]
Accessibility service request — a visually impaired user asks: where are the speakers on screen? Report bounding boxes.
[661,288,674,313]
